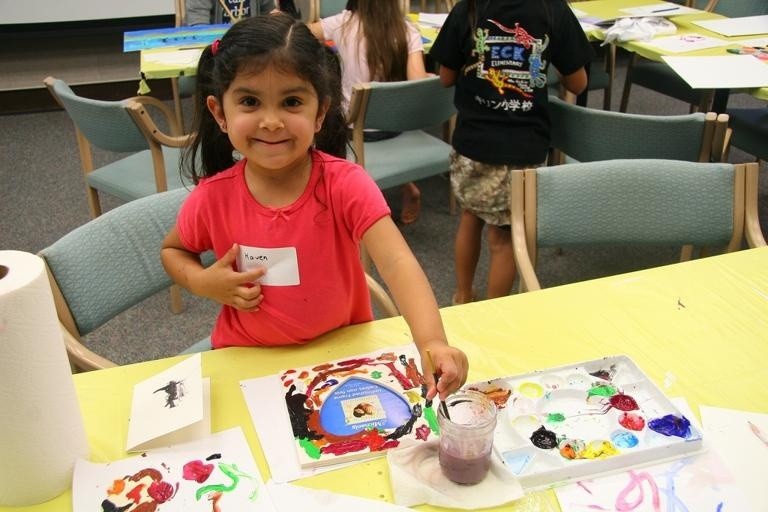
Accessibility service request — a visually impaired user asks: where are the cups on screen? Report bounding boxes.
[435,389,497,483]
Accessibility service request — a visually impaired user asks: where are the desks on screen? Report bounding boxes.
[1,244,768,512]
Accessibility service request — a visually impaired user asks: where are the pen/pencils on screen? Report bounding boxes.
[652,7,680,13]
[178,46,204,51]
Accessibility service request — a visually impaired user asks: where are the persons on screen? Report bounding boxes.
[424,0,595,306]
[158,13,470,404]
[303,1,440,225]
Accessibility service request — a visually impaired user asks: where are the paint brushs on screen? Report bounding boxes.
[426,349,453,419]
